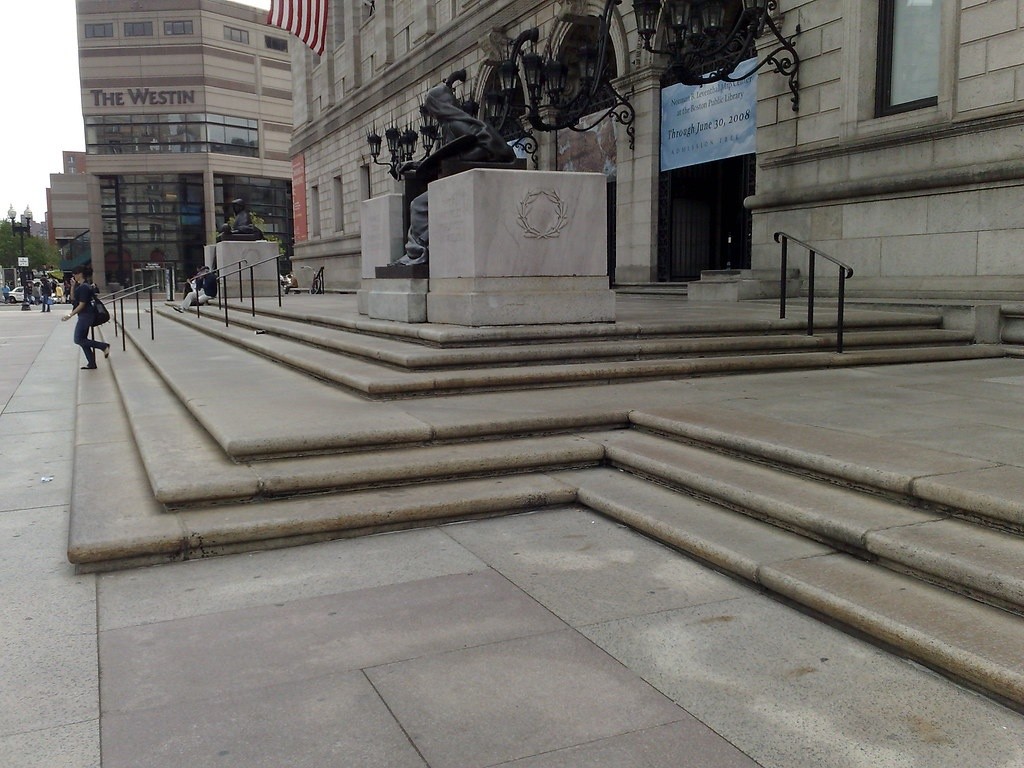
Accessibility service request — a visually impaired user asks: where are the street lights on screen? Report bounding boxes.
[7,202,33,310]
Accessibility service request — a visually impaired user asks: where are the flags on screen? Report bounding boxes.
[267,0,328,58]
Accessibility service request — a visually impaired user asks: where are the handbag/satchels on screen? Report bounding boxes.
[47,298,53,304]
[90,299,110,327]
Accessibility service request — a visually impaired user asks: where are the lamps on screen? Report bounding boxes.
[416,72,538,170]
[365,109,439,182]
[494,0,635,153]
[631,0,800,112]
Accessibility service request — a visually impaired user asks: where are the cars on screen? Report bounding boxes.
[1,286,44,304]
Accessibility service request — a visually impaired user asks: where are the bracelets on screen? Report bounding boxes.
[68,314,71,318]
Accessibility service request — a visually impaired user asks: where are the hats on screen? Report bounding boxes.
[200,266,209,271]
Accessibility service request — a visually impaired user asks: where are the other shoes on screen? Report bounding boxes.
[103,344,110,358]
[173,306,184,313]
[80,366,97,369]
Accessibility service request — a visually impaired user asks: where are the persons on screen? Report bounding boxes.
[17,268,100,312]
[280,274,298,295]
[173,266,217,313]
[2,282,10,304]
[62,272,110,369]
[391,83,512,267]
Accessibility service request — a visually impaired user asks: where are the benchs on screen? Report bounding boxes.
[284,288,313,294]
